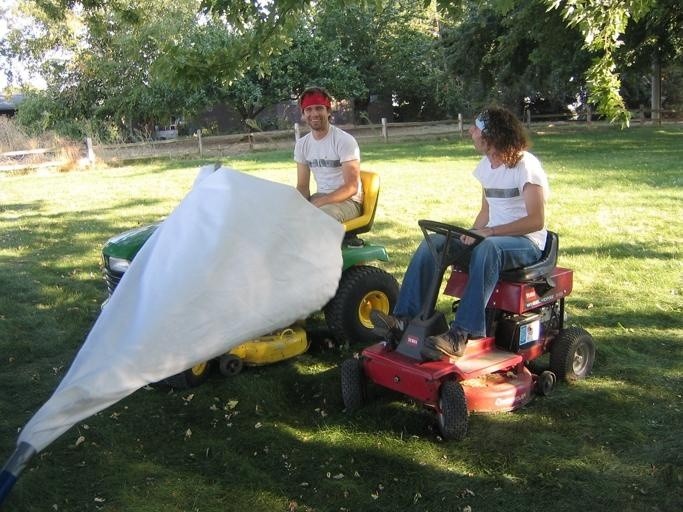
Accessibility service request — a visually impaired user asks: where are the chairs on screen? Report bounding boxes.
[341,169,380,235]
[452,230,558,281]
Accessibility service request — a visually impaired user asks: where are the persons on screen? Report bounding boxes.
[291,86,366,229]
[367,105,551,355]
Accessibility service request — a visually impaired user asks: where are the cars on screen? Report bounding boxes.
[154,125,179,140]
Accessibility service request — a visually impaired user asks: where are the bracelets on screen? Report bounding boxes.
[489,226,495,237]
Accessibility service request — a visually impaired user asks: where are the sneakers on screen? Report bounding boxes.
[423,324,472,359]
[367,309,408,347]
